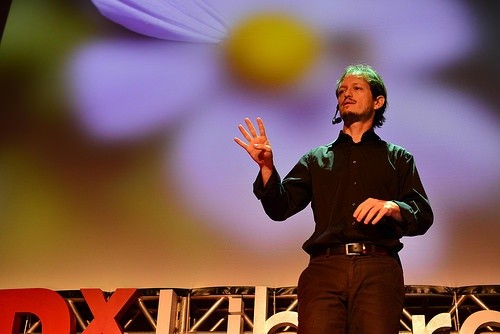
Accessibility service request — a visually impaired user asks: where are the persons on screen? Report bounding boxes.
[234,65,434,334]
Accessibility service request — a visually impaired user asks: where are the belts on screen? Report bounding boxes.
[310,241,403,257]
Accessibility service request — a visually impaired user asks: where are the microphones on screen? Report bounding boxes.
[332,104,342,124]
